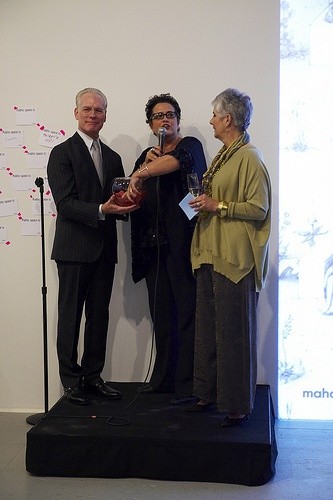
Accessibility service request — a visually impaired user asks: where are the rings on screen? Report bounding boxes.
[196,202,201,206]
[151,148,154,152]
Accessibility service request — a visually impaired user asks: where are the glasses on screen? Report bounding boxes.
[149,112,178,122]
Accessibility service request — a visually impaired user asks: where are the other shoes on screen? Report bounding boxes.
[186,402,212,412]
[219,415,246,427]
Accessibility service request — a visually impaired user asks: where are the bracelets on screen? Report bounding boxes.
[143,163,152,176]
[218,201,228,218]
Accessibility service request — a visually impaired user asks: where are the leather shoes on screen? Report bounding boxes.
[64,385,89,405]
[85,382,121,397]
[171,394,194,405]
[137,385,153,394]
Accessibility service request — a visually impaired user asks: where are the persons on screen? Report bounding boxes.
[121,93,207,404]
[47,87,140,406]
[182,88,272,428]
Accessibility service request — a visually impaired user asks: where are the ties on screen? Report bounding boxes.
[92,142,104,189]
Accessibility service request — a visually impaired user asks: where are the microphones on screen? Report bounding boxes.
[157,128,167,156]
